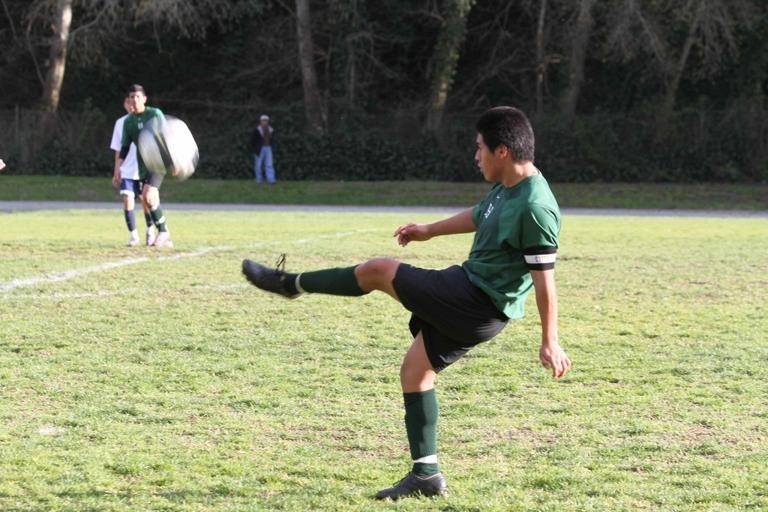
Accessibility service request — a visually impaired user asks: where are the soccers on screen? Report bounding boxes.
[137,117,197,175]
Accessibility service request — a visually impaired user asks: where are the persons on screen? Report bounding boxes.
[114,83,176,246]
[109,95,155,246]
[251,113,277,185]
[240,105,574,501]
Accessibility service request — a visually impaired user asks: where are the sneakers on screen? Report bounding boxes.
[128,226,174,249]
[242,258,303,300]
[375,470,448,502]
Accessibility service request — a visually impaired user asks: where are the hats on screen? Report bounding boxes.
[259,115,269,121]
